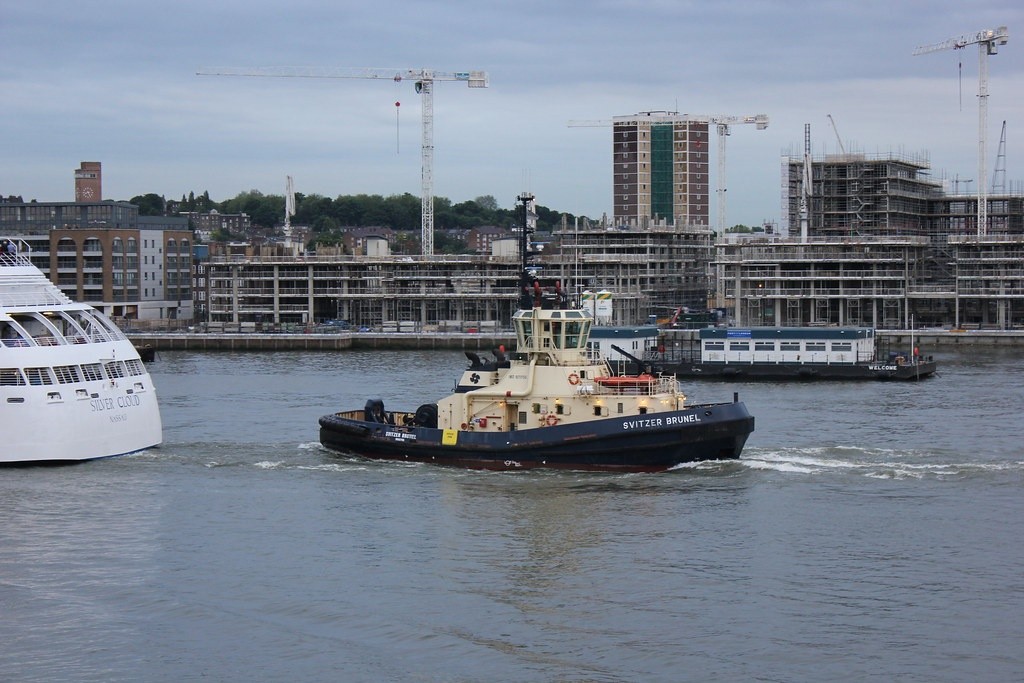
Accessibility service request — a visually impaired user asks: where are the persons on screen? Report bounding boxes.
[914,345,920,362]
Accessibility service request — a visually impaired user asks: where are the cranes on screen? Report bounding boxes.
[195,63,490,255]
[911,25,1009,235]
[566,111,768,310]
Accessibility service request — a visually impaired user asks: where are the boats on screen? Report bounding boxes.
[513,191,937,381]
[316,278,755,471]
[0,233,164,464]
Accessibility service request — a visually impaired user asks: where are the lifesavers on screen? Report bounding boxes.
[545,414,558,427]
[568,373,581,386]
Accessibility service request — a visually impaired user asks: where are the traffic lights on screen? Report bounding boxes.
[756,282,763,295]
